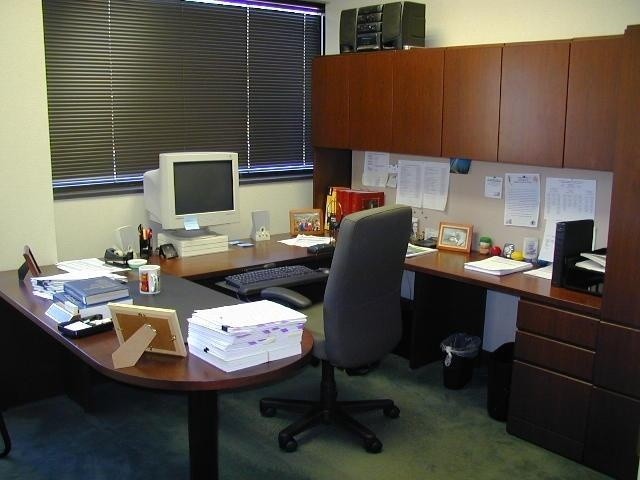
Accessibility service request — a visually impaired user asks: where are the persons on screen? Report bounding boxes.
[413,222,417,235]
[527,240,536,255]
[294,217,320,231]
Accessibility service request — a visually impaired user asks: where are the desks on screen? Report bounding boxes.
[0,258,315,480]
[136,223,602,425]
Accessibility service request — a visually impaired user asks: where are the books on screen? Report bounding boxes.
[324,183,384,232]
[572,252,607,274]
[463,255,533,276]
[405,242,439,258]
[183,299,308,373]
[28,257,134,318]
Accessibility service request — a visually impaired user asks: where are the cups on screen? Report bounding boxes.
[138,264,161,296]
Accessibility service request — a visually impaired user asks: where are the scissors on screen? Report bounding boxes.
[138,224,143,233]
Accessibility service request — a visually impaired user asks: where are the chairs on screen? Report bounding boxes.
[259,203,413,456]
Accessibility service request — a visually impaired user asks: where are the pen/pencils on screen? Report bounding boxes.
[142,228,152,240]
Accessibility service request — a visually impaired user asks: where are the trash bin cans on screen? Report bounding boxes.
[441,333,481,390]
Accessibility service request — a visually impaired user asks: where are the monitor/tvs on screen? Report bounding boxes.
[143,151,239,236]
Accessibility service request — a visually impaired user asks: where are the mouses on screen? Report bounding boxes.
[315,267,330,274]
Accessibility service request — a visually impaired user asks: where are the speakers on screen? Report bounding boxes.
[339,8,356,53]
[381,1,425,49]
[488,342,514,422]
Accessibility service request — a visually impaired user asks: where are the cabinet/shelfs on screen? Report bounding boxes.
[506,293,640,480]
[442,43,502,162]
[392,49,444,156]
[563,37,625,171]
[309,54,351,148]
[497,39,571,167]
[351,51,392,150]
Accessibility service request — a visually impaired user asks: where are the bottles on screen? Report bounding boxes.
[522,237,539,262]
[480,237,492,254]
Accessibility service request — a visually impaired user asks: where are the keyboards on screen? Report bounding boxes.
[224,265,314,288]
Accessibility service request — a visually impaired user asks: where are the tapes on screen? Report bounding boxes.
[114,251,123,257]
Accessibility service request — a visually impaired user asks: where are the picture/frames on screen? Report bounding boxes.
[436,221,474,255]
[290,208,325,239]
[25,245,43,275]
[105,304,187,355]
